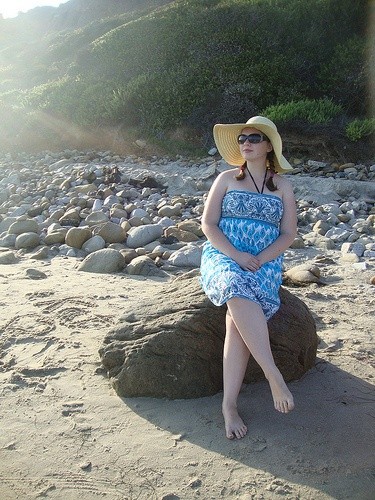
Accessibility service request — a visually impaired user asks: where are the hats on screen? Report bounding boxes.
[212,116,293,174]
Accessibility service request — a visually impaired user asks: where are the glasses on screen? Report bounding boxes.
[237,134,269,144]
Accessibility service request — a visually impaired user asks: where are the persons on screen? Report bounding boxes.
[199,115,298,439]
